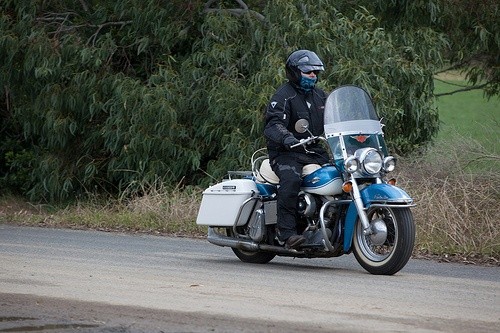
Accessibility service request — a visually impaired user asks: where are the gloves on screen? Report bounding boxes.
[282,135,300,150]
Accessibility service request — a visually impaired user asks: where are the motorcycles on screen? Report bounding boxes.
[195,84,416,278]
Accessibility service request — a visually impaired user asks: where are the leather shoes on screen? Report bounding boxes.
[286,235,305,249]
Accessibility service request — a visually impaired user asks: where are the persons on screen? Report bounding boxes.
[264,50,331,248]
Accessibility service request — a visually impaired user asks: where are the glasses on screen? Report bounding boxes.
[301,70,319,75]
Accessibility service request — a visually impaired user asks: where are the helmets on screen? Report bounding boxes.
[286,50,323,87]
